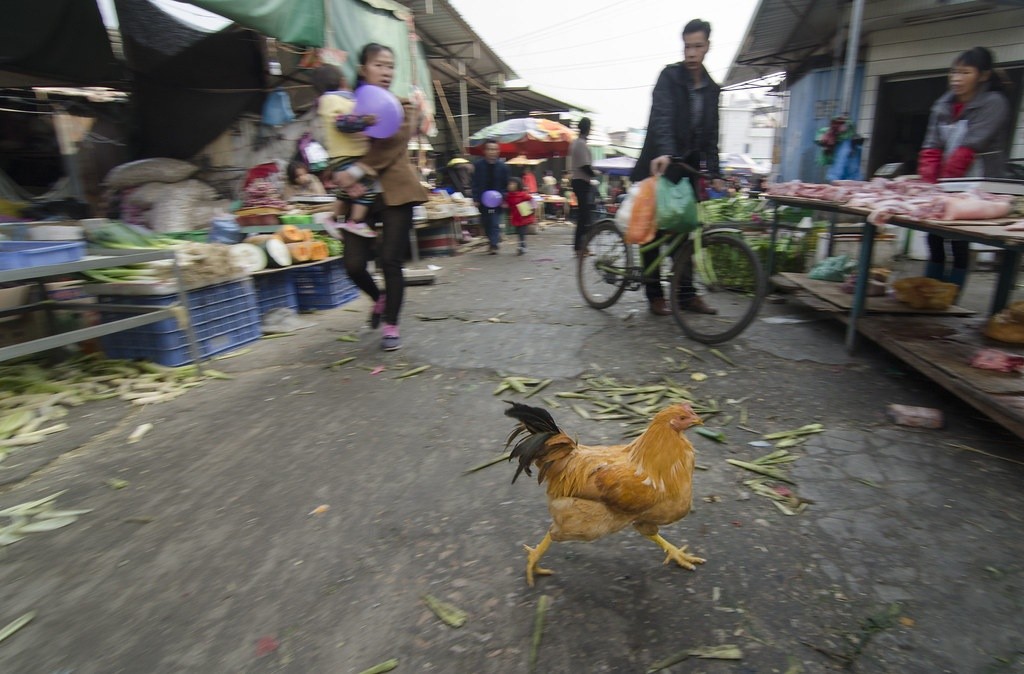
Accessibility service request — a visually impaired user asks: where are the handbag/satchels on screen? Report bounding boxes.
[615,173,700,245]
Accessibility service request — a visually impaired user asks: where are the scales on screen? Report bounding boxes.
[400,205,436,286]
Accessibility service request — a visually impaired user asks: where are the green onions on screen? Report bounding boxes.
[78,221,236,286]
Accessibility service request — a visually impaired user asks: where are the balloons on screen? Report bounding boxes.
[353,84,404,140]
[481,190,503,207]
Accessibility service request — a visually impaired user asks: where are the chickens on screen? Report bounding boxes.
[501,399,708,588]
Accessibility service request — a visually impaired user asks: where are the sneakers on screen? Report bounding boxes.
[381,322,400,350]
[371,293,387,329]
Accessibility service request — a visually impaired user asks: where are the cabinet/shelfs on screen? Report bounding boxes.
[756,192,1024,438]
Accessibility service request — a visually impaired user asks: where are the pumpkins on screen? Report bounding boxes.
[267,223,328,263]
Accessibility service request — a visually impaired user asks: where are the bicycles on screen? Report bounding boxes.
[574,156,768,344]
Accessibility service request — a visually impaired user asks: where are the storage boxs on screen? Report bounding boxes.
[0,239,360,367]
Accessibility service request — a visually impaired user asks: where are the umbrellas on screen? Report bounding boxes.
[463,117,577,158]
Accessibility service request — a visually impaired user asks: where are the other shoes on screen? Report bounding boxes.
[680,296,719,314]
[346,221,379,237]
[489,245,498,254]
[649,298,675,315]
[323,217,345,242]
[518,246,528,255]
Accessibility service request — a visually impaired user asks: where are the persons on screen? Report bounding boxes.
[522,166,538,192]
[501,177,537,252]
[313,63,380,241]
[471,141,508,253]
[570,117,595,256]
[630,19,726,314]
[918,47,1013,302]
[280,160,327,202]
[540,170,559,219]
[343,42,430,352]
[452,158,476,198]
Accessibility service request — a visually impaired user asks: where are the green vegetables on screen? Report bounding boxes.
[314,233,344,256]
